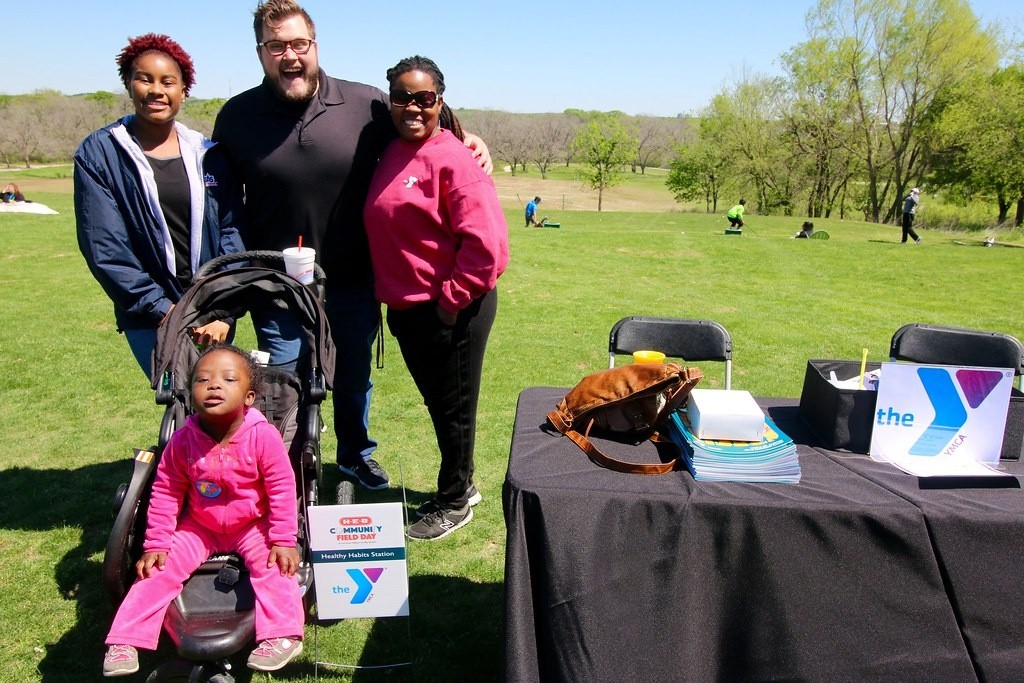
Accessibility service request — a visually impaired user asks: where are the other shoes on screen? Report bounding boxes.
[916,237,921,244]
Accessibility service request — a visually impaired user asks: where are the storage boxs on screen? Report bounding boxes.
[799,357,1024,463]
[305,503,410,620]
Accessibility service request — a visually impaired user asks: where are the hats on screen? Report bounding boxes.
[911,188,920,194]
[536,196,541,202]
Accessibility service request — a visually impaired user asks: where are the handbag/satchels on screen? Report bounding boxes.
[547,363,703,474]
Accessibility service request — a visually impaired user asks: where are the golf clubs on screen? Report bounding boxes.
[743,222,759,234]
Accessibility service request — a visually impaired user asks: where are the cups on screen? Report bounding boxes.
[283,247,315,285]
[633,351,665,366]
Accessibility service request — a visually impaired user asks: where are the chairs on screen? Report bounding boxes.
[608,317,732,390]
[890,324,1024,391]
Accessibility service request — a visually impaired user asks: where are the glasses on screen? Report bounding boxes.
[390,89,439,108]
[259,38,314,56]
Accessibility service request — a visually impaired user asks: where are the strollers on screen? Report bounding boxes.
[101,250,338,683]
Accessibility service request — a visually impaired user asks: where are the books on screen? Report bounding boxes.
[667,407,803,484]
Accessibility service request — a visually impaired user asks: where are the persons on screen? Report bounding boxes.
[899,187,921,243]
[74,34,252,383]
[795,222,814,238]
[0,184,25,202]
[362,56,509,540]
[211,0,494,489]
[727,199,745,228]
[103,344,304,675]
[525,196,542,227]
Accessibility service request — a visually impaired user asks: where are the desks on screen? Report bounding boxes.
[501,387,1024,681]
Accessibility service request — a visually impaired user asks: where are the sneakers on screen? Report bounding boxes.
[340,457,390,490]
[247,638,304,672]
[103,644,140,676]
[405,504,473,541]
[416,485,482,516]
